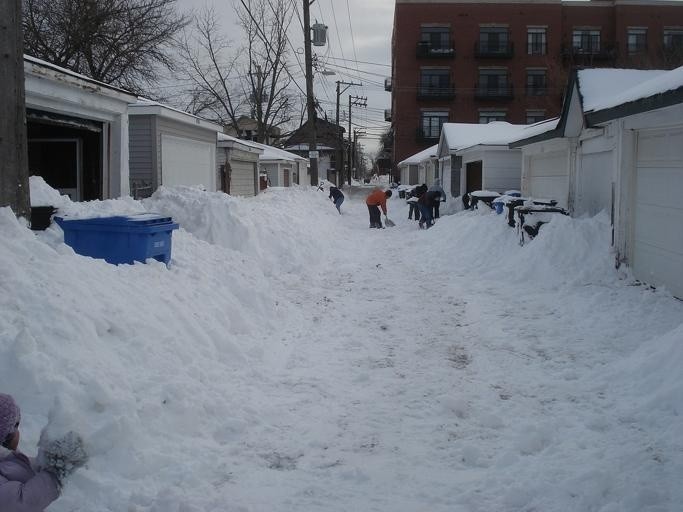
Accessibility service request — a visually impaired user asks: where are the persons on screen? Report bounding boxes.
[427,178,446,219]
[328,185,344,215]
[365,189,392,229]
[416,192,442,231]
[405,183,428,222]
[2,395,88,511]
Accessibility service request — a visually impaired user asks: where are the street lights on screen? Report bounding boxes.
[312,70,336,76]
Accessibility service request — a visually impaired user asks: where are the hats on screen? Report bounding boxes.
[386,190,392,196]
[0,393,20,443]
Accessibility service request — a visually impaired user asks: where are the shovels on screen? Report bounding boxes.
[385,215,395,227]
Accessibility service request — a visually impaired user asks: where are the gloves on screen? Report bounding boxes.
[45,433,89,481]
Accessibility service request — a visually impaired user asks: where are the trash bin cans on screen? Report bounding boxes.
[468,193,568,246]
[398,191,405,198]
[30,207,180,267]
